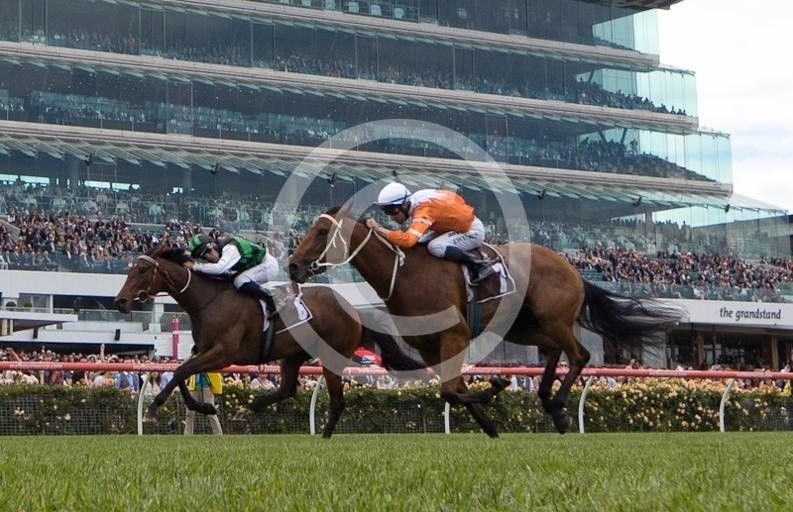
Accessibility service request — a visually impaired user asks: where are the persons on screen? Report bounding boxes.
[0,0,793,394]
[176,342,224,436]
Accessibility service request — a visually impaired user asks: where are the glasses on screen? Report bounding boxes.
[201,246,212,260]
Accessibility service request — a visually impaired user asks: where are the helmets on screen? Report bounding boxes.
[189,234,210,259]
[372,181,413,206]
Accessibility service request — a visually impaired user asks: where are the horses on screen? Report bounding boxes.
[113,244,436,439]
[287,196,680,439]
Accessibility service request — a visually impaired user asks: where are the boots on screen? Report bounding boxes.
[238,279,287,321]
[444,245,495,283]
